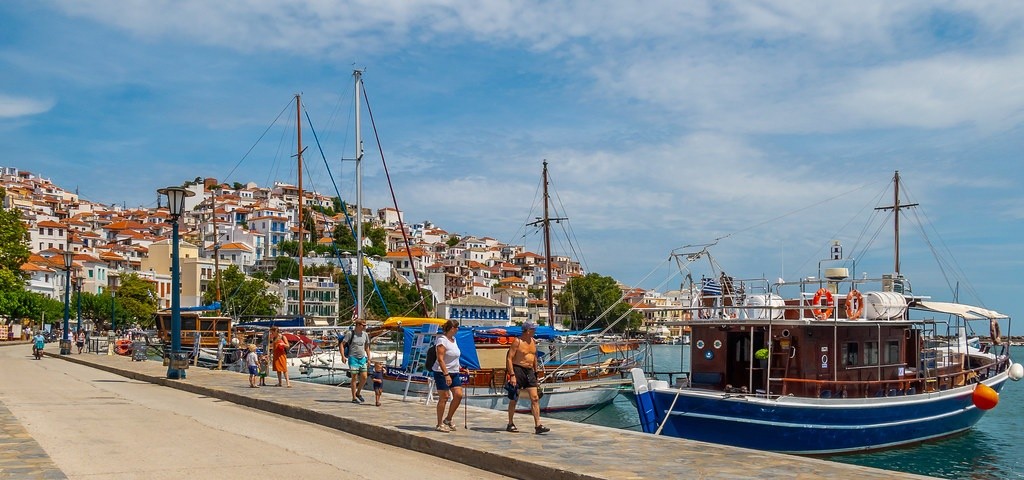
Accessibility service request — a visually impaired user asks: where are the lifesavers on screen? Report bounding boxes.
[990,319,1001,345]
[920,335,924,352]
[844,289,864,321]
[812,288,834,320]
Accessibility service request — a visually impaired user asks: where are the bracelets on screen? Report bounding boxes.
[510,374,515,376]
[444,373,449,376]
[459,368,464,372]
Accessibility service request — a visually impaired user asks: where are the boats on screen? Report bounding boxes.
[615,170,1023,457]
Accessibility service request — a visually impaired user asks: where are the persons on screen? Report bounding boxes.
[76,329,85,354]
[24,326,30,341]
[30,331,45,356]
[68,329,73,352]
[231,324,236,332]
[340,319,370,403]
[258,355,269,386]
[432,320,469,432]
[269,326,292,388]
[506,320,550,434]
[370,361,386,406]
[246,344,260,388]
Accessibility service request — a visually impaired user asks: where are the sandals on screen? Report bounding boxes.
[435,424,450,432]
[444,419,457,431]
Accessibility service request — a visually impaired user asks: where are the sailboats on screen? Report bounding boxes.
[131,66,654,413]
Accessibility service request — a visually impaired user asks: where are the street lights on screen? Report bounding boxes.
[74,277,86,337]
[109,288,117,332]
[58,251,78,354]
[157,186,197,380]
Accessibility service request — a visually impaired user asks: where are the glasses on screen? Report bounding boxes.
[361,324,366,327]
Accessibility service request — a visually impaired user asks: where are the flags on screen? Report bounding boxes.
[703,278,721,294]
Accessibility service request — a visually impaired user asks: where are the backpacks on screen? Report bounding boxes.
[426,336,446,370]
[338,329,355,358]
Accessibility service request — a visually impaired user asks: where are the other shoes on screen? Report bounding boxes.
[262,383,267,386]
[275,383,282,386]
[353,398,361,403]
[258,383,261,386]
[507,424,519,433]
[359,395,366,402]
[252,385,260,388]
[535,424,550,433]
[250,384,254,387]
[287,383,292,387]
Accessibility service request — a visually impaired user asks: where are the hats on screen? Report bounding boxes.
[521,320,538,332]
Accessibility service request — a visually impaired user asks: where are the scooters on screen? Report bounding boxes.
[32,342,43,360]
[43,333,58,344]
